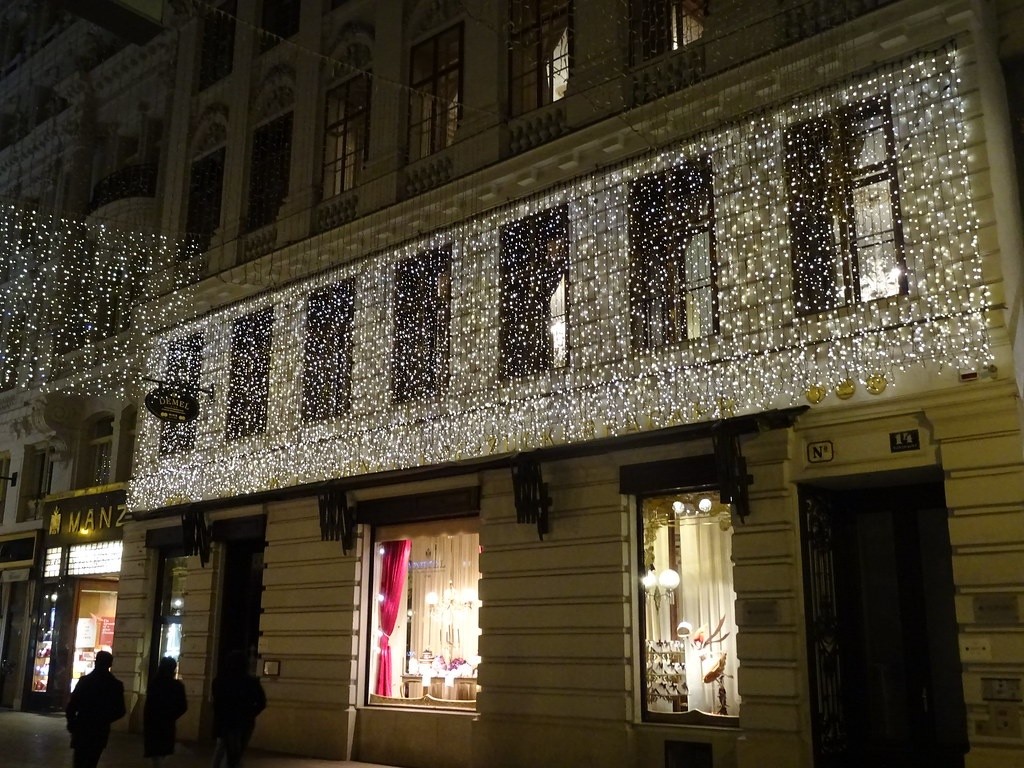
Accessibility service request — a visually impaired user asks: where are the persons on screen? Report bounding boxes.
[65,651,126,768]
[142,658,187,768]
[209,648,266,768]
